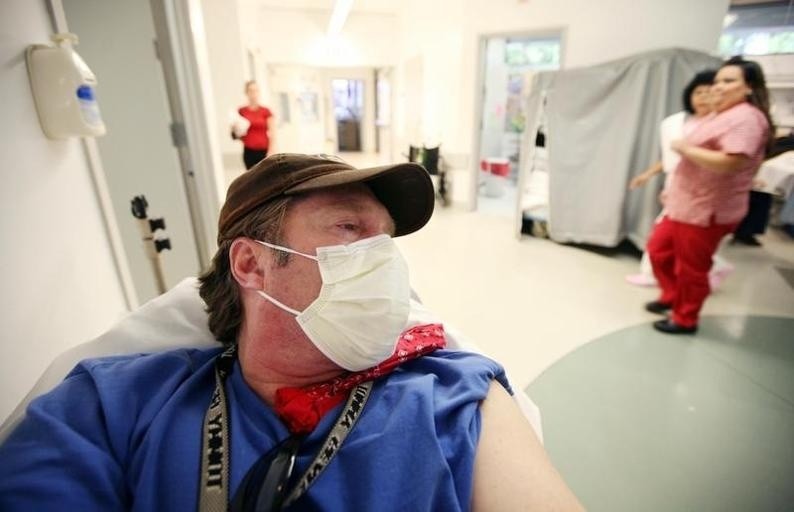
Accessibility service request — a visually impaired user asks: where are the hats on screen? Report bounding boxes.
[217,151,435,243]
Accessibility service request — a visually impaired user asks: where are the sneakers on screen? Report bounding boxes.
[647,301,698,334]
[710,266,732,293]
[628,274,654,286]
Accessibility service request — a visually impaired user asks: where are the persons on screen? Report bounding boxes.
[231,81,274,170]
[0,152,586,512]
[627,53,794,333]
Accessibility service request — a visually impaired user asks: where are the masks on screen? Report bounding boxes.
[246,235,413,375]
